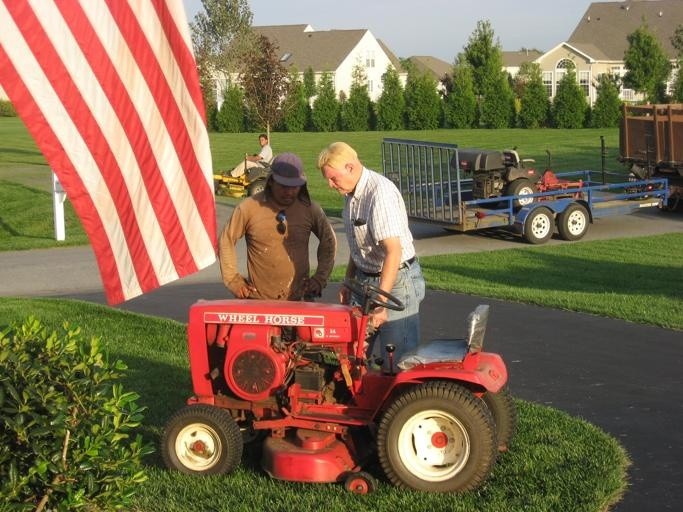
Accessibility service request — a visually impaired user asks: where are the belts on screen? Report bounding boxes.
[363,257,415,276]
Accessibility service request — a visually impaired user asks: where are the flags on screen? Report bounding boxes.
[0,1,220,307]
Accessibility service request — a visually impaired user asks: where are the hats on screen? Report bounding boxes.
[272,152,306,190]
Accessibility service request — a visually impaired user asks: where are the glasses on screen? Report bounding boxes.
[277,210,286,235]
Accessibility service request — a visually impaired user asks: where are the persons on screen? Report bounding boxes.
[215,153,340,301]
[315,139,432,474]
[222,134,277,179]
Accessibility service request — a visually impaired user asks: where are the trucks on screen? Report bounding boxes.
[617,102,682,211]
